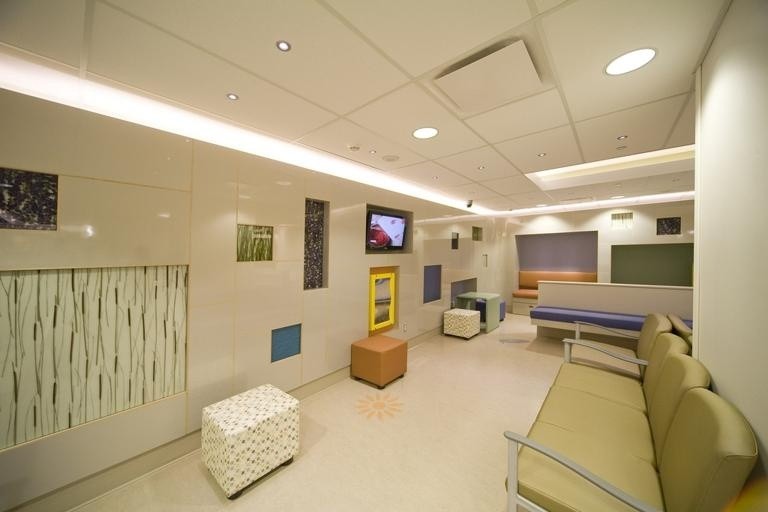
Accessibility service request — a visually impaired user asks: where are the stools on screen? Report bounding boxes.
[444,308,480,340]
[200,384,300,500]
[352,335,407,389]
[476,300,505,322]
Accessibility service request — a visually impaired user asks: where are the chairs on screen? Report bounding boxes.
[564,313,671,378]
[686,336,693,346]
[667,313,692,336]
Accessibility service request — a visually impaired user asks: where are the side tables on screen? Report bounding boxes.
[456,291,500,333]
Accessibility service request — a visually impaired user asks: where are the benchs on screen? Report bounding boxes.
[505,333,757,512]
[512,271,596,315]
[530,281,693,350]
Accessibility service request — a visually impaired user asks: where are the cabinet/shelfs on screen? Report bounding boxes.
[369,273,395,331]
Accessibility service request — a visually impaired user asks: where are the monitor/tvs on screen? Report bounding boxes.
[366,210,408,250]
